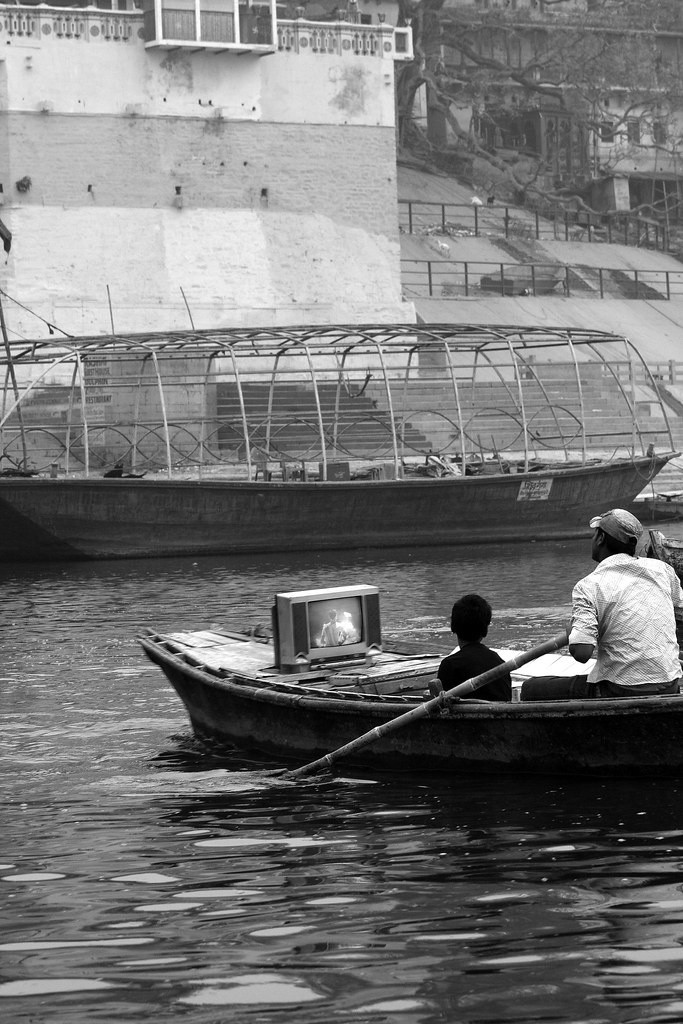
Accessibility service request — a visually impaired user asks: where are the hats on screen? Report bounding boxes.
[590,508,644,544]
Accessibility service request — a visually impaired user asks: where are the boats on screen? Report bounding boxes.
[640,528,683,642]
[0,315,683,559]
[137,623,683,771]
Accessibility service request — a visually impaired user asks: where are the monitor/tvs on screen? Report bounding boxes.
[271,583,384,677]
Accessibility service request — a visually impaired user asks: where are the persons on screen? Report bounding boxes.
[321,609,359,646]
[437,594,512,704]
[520,508,683,702]
